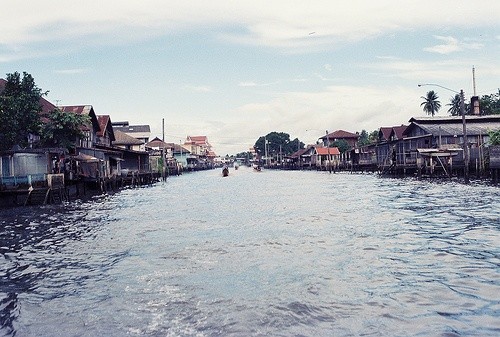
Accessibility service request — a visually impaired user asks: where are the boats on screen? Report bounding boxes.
[222,167,229,176]
[254,166,262,173]
